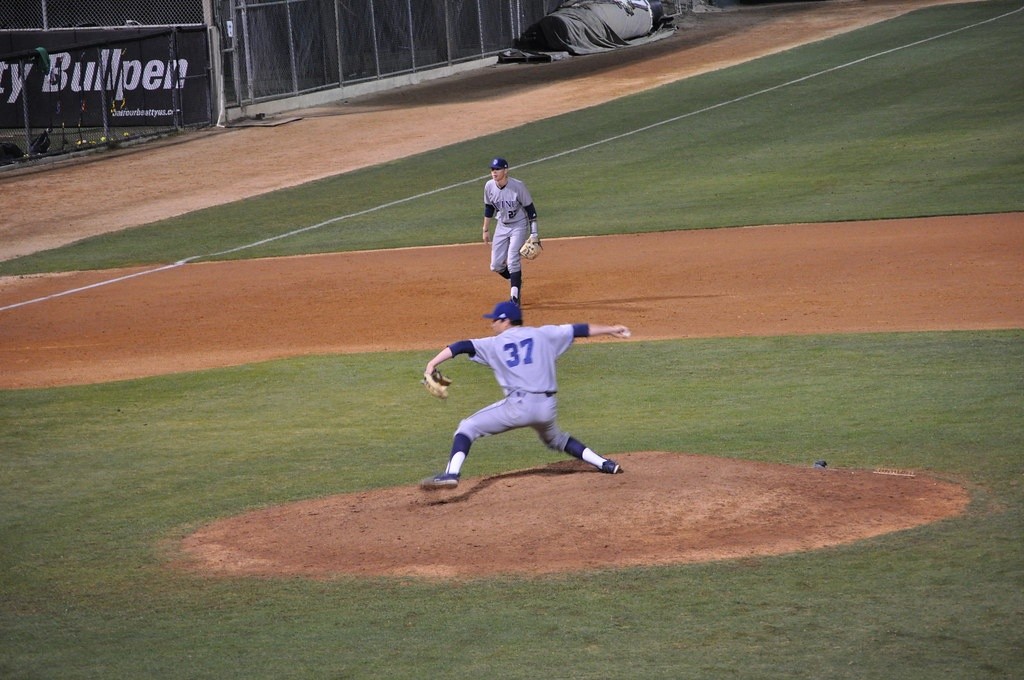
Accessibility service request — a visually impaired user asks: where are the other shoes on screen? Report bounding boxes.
[509,296,520,306]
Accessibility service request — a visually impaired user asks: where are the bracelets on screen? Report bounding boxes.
[485,230,488,232]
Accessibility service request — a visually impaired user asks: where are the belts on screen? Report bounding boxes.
[546,391,553,396]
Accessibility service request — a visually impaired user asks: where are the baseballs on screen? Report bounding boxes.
[623,330,632,339]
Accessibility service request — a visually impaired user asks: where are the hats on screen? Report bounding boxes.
[483,302,521,321]
[489,159,509,170]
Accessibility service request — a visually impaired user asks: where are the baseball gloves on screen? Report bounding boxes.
[519,236,542,260]
[422,372,452,399]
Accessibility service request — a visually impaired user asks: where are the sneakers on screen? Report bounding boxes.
[598,458,620,474]
[421,472,461,488]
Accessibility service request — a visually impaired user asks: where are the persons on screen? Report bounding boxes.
[482,157,542,306]
[417,302,631,489]
